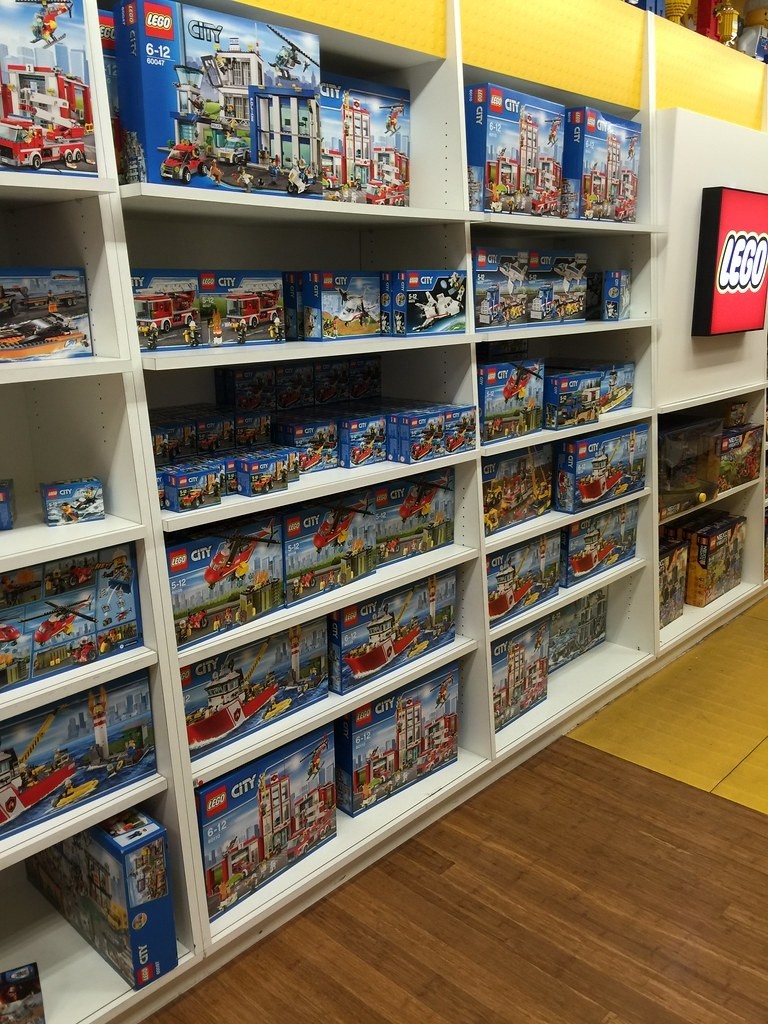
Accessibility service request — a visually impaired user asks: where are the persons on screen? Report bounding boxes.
[738,7,768,63]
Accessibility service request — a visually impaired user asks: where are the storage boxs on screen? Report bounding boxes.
[133,270,467,353]
[474,245,631,333]
[179,567,456,763]
[659,508,747,631]
[194,720,336,924]
[40,476,105,527]
[98,0,412,208]
[24,806,178,992]
[0,962,46,1024]
[334,662,459,818]
[490,586,608,733]
[1,478,17,531]
[481,422,649,536]
[466,82,641,223]
[475,338,635,446]
[149,352,477,513]
[0,667,158,839]
[0,541,144,694]
[658,400,764,521]
[1,0,97,178]
[486,500,639,629]
[164,467,456,653]
[1,267,92,363]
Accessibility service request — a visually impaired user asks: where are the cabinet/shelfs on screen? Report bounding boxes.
[0,0,768,1024]
